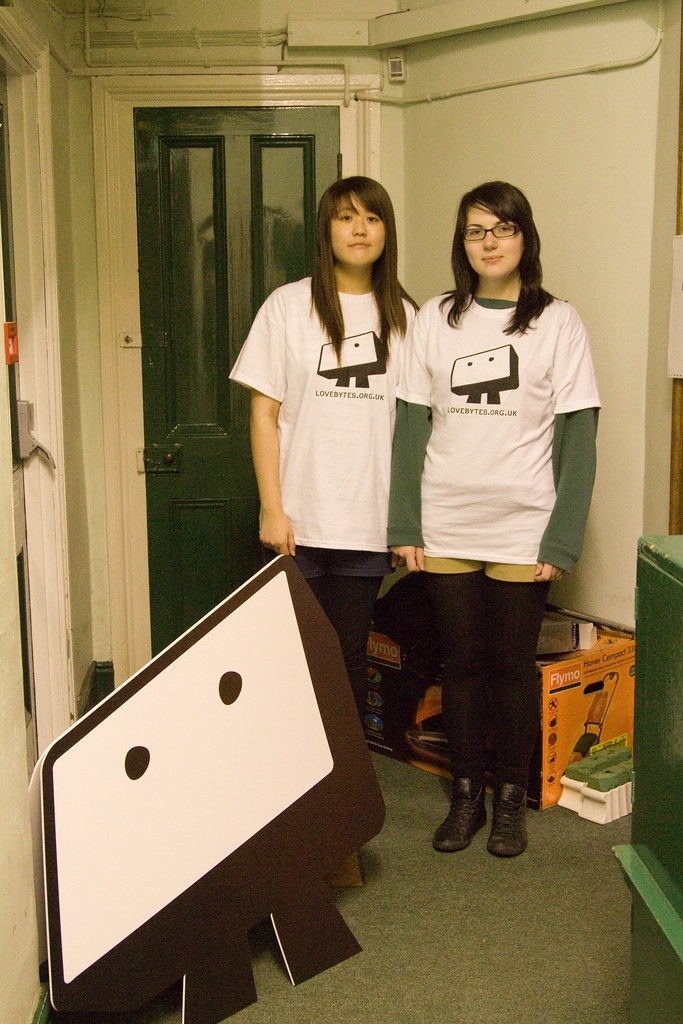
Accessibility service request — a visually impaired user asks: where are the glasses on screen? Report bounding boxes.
[460,224,520,241]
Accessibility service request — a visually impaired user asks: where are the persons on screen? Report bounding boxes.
[226,176,417,731]
[387,180,603,857]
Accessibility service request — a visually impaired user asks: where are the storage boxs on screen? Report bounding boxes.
[362,612,636,812]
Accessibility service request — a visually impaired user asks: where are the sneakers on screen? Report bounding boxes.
[488,782,529,856]
[432,779,487,851]
